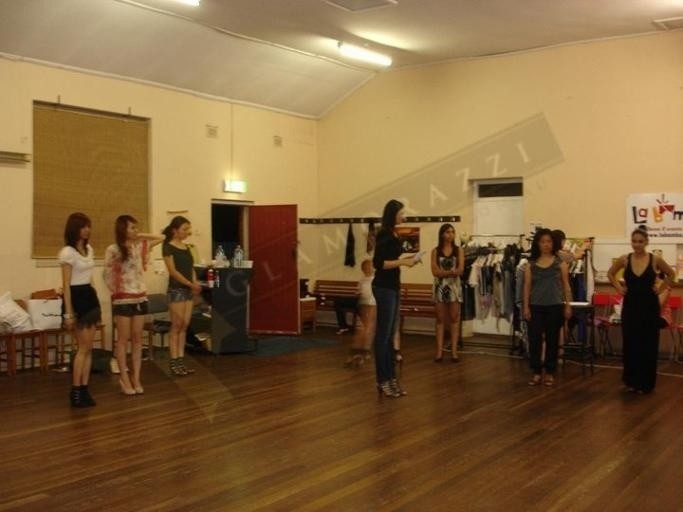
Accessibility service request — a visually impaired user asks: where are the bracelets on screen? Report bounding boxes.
[186,281,191,286]
[451,269,456,275]
[63,313,74,320]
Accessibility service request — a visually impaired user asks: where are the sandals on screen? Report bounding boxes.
[527,373,542,386]
[542,372,557,390]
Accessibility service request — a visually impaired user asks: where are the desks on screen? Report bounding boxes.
[299,297,318,336]
[511,304,595,369]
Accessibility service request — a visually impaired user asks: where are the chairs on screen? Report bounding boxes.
[0,283,173,380]
[572,292,682,366]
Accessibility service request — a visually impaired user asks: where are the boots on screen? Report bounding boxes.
[68,385,89,409]
[80,384,96,407]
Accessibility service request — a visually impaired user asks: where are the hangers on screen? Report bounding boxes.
[464,236,581,256]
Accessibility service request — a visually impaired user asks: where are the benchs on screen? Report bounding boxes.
[310,276,459,346]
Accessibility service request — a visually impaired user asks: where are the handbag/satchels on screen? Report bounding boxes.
[27,295,63,329]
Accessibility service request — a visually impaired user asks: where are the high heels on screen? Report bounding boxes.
[130,376,145,394]
[392,349,404,371]
[120,379,136,395]
[375,378,408,398]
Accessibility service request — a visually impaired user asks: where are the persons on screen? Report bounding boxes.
[162,216,202,377]
[104,215,167,395]
[333,259,404,370]
[431,223,465,363]
[371,200,423,398]
[59,212,102,408]
[552,230,592,265]
[522,228,572,387]
[607,225,675,395]
[656,272,672,329]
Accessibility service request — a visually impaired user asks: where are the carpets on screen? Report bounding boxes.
[245,335,341,357]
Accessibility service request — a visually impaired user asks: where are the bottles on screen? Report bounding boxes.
[207,245,244,288]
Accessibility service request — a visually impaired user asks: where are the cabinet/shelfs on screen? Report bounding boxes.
[189,263,258,356]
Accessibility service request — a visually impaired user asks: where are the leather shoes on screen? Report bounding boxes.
[177,357,196,374]
[451,351,459,363]
[434,350,444,363]
[167,358,189,375]
[618,383,645,396]
